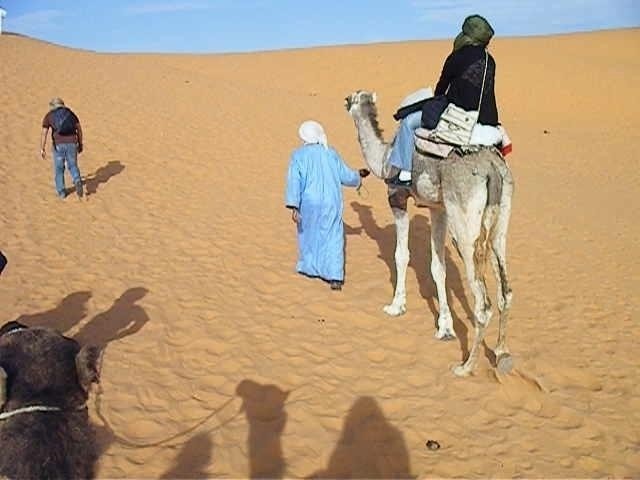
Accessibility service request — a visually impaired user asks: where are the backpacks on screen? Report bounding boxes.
[51,108,76,134]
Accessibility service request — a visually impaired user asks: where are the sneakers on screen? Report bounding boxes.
[331,280,341,289]
[385,172,412,186]
[75,178,83,196]
[59,189,65,198]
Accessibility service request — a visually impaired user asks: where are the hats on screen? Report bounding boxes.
[49,98,64,105]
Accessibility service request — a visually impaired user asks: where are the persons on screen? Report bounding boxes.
[39,94,85,200]
[284,118,372,291]
[384,12,512,190]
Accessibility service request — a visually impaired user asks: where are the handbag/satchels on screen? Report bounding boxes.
[436,103,479,146]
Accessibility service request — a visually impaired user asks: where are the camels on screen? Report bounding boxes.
[344,89,514,379]
[0,321,101,480]
